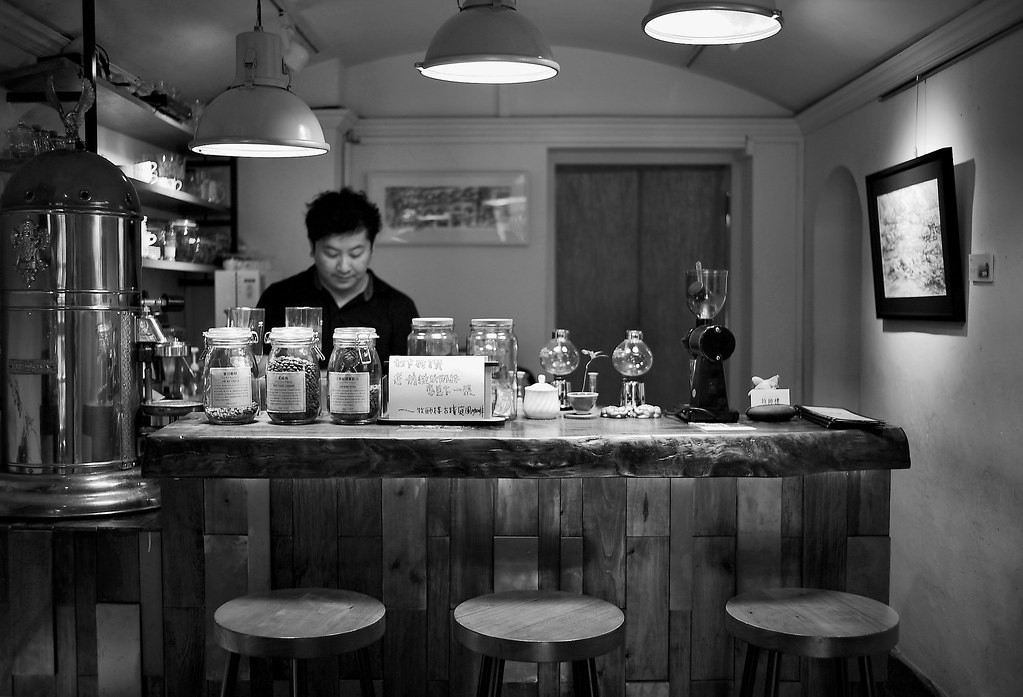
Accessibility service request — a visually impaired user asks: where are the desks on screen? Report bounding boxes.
[152,412,913,697]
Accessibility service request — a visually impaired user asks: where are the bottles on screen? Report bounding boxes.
[162,218,229,261]
[164,154,228,203]
[4,120,66,157]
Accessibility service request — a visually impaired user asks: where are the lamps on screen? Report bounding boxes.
[639,0,785,46]
[187,0,332,159]
[412,0,561,85]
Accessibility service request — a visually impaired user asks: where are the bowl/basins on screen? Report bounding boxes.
[566,390,598,412]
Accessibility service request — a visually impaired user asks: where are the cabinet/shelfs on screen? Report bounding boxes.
[23,53,239,340]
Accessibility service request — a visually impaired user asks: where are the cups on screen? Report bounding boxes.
[409,317,459,356]
[179,362,203,403]
[468,318,518,419]
[225,304,264,365]
[523,375,560,420]
[118,154,158,185]
[286,304,322,357]
[159,175,183,194]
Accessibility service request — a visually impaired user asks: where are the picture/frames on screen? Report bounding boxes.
[365,169,531,246]
[864,147,966,324]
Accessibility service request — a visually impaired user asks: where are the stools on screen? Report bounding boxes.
[725,585,901,697]
[214,585,388,697]
[452,588,626,697]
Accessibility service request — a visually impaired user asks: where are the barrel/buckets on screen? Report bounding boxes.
[1,148,161,518]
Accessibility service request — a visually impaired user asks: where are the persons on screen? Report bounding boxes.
[250,182,420,370]
